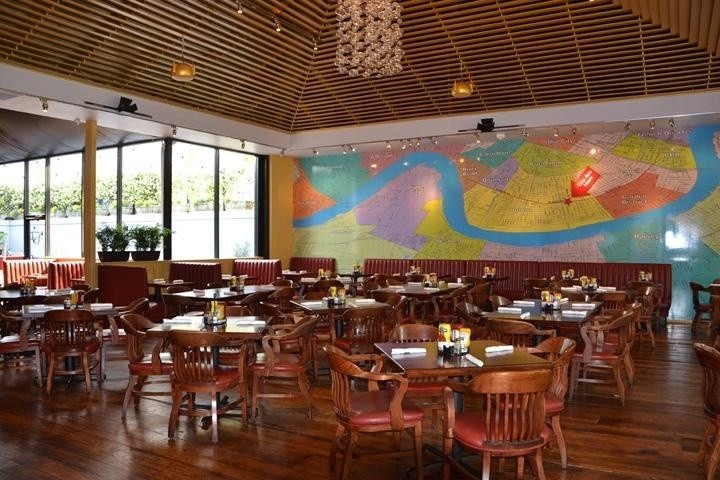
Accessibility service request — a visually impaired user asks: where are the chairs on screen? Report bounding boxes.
[224,305,251,316]
[336,308,386,356]
[364,277,377,288]
[485,336,575,474]
[460,275,477,284]
[708,284,720,347]
[488,295,510,311]
[588,302,641,391]
[43,309,105,394]
[629,283,663,349]
[522,277,550,300]
[487,319,556,351]
[455,302,487,335]
[259,300,310,324]
[267,279,294,286]
[438,366,554,479]
[322,345,424,480]
[304,292,331,299]
[693,341,720,480]
[102,296,149,363]
[374,291,399,306]
[690,282,714,336]
[437,275,454,282]
[408,274,425,282]
[0,305,44,388]
[166,328,252,445]
[386,323,461,440]
[439,283,473,311]
[410,300,440,323]
[374,274,387,286]
[251,318,317,424]
[241,291,265,313]
[313,279,343,290]
[118,313,194,417]
[569,308,638,408]
[266,286,303,301]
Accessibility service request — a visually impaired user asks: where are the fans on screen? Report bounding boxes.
[458,118,526,132]
[84,94,152,118]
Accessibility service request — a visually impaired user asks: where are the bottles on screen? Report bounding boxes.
[318,269,330,280]
[211,301,226,319]
[21,278,36,295]
[231,276,245,291]
[460,328,471,347]
[204,302,210,320]
[553,297,558,310]
[638,271,650,282]
[213,312,217,323]
[581,278,597,289]
[438,323,451,343]
[425,273,437,288]
[460,338,465,355]
[64,291,84,307]
[455,339,461,355]
[322,286,346,303]
[557,299,561,310]
[208,312,212,323]
[451,324,464,341]
[485,267,496,276]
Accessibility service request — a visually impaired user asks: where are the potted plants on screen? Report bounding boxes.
[96,226,130,262]
[127,224,176,261]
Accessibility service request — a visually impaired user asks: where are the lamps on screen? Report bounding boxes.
[170,60,195,82]
[451,80,475,98]
[334,0,405,78]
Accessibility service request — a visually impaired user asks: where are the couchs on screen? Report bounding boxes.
[411,260,465,280]
[538,262,604,284]
[3,258,49,287]
[48,261,85,289]
[98,263,159,321]
[363,258,412,279]
[168,262,222,318]
[231,260,284,286]
[603,263,672,313]
[289,256,336,287]
[466,260,538,297]
